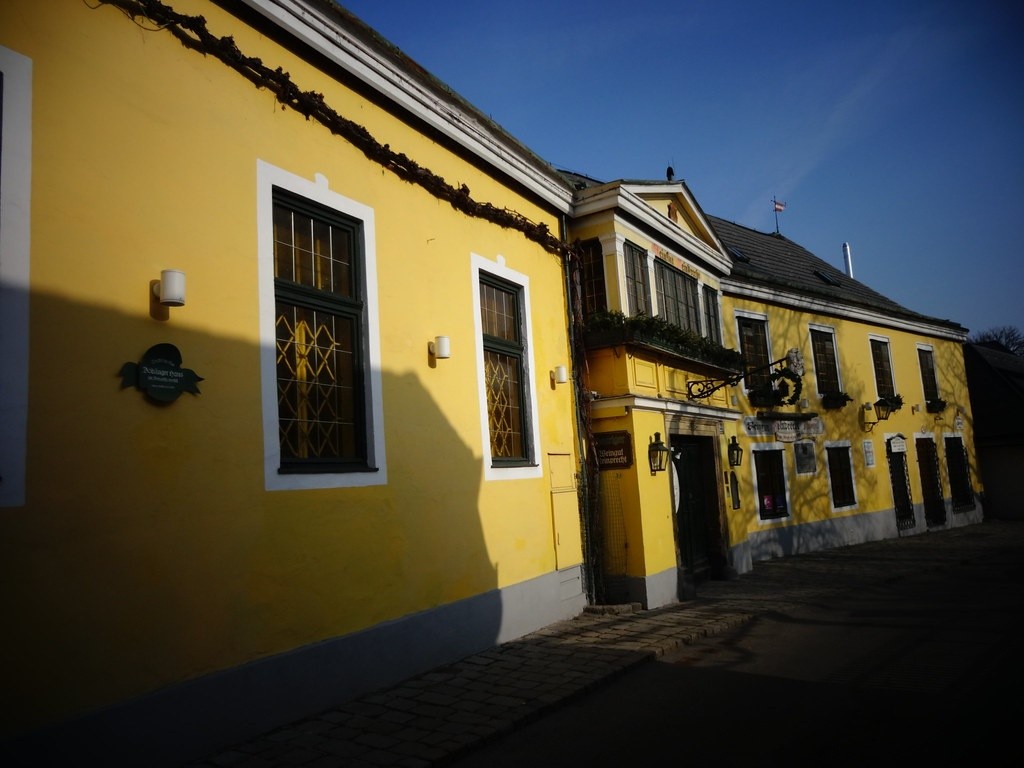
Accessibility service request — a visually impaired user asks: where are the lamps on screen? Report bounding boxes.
[728,436,743,466]
[649,432,669,471]
[873,399,893,420]
